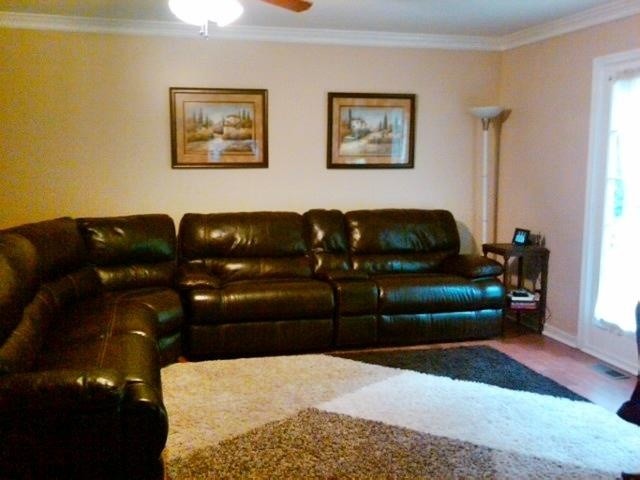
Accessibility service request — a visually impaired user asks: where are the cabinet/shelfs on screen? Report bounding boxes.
[482,240,550,334]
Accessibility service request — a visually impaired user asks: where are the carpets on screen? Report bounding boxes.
[158,342,639,480]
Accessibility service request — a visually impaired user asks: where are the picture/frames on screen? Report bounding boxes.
[511,226,530,246]
[325,89,418,171]
[168,85,272,171]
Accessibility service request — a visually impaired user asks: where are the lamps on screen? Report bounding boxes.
[465,102,507,259]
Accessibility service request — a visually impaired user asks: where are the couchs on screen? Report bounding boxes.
[1,214,181,477]
[181,208,508,357]
